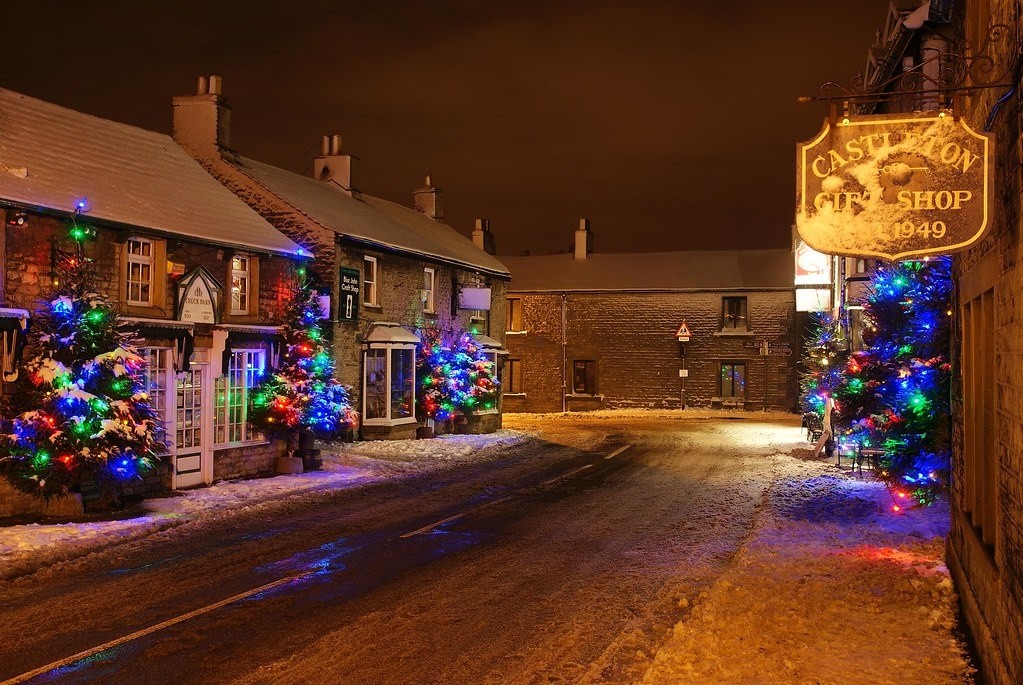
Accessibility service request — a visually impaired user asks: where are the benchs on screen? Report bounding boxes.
[851,439,887,478]
[806,411,823,443]
